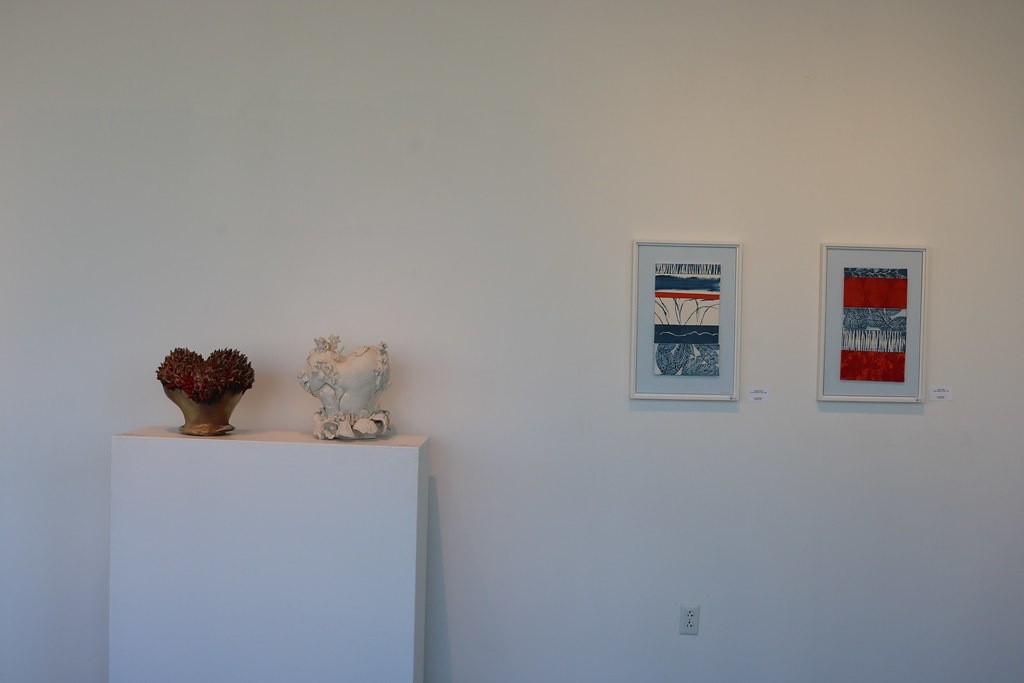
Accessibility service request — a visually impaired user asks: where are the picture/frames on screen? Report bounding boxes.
[629,237,742,403]
[817,244,929,403]
